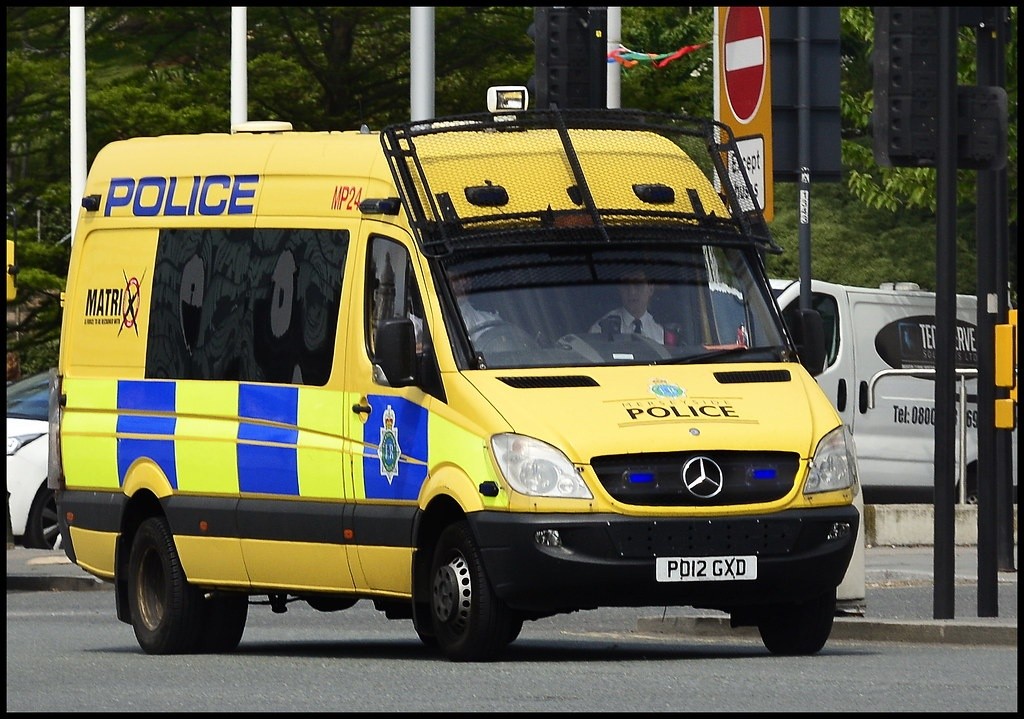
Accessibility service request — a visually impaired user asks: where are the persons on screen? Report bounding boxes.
[409,257,504,353]
[582,260,667,350]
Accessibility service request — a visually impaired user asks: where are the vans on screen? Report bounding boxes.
[48,85,864,662]
[766,276,1018,504]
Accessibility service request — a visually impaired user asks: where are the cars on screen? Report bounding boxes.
[5,364,64,552]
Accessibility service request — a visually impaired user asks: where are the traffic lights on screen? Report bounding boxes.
[6,241,19,303]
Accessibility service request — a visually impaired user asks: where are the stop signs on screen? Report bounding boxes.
[721,6,770,125]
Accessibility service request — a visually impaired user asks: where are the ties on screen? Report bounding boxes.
[632,319,643,334]
